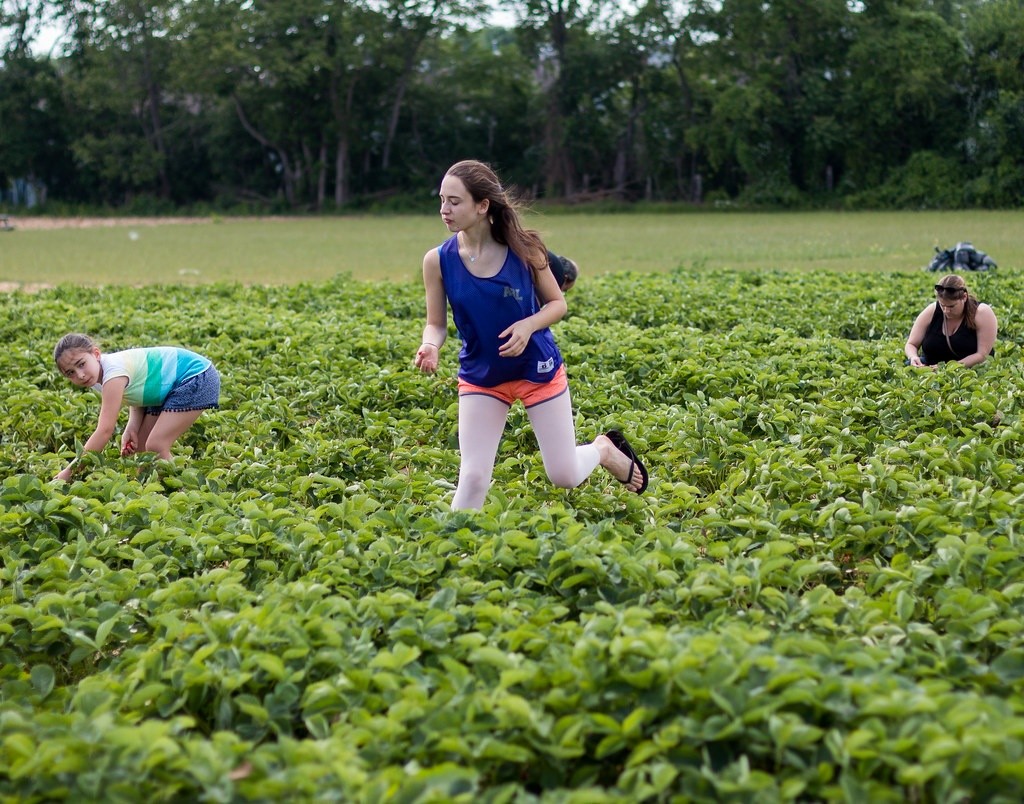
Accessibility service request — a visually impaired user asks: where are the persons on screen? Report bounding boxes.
[415,159,649,526]
[903,275,997,370]
[52,334,221,486]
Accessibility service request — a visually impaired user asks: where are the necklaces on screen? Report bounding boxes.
[462,232,487,262]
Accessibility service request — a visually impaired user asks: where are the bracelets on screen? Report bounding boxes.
[423,343,439,349]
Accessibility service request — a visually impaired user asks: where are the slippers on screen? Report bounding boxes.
[606,430,648,495]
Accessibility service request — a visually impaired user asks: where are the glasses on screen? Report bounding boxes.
[934,285,965,294]
